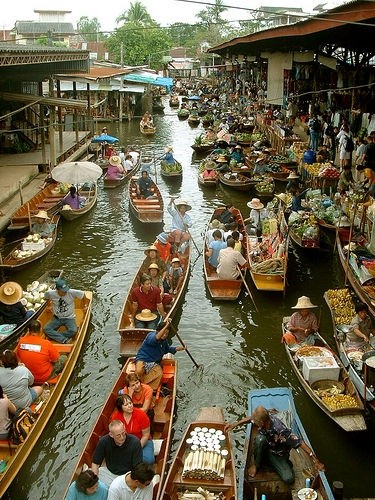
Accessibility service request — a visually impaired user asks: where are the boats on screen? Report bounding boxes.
[139,119,156,135]
[160,407,238,500]
[60,180,98,222]
[282,316,368,433]
[324,289,375,411]
[7,181,75,235]
[96,148,118,169]
[169,80,267,149]
[0,215,60,273]
[244,156,296,183]
[202,206,249,301]
[101,149,142,189]
[238,388,336,500]
[279,194,320,249]
[261,150,298,172]
[335,228,375,319]
[0,269,64,343]
[216,166,256,192]
[247,208,286,292]
[197,156,220,187]
[61,357,178,500]
[251,172,276,200]
[161,157,183,178]
[116,231,192,357]
[229,156,253,177]
[0,289,93,500]
[301,194,352,233]
[128,176,164,224]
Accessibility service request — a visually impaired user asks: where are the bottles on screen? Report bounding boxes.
[306,478,311,488]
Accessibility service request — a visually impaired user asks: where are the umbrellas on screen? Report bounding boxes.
[90,135,118,146]
[51,159,102,193]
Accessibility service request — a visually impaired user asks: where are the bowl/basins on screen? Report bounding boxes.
[336,324,352,334]
[287,343,301,352]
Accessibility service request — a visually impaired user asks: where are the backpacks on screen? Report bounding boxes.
[343,134,354,152]
[7,407,36,456]
[312,120,320,131]
[326,123,334,136]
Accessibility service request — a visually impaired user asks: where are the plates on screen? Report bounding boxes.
[297,487,317,500]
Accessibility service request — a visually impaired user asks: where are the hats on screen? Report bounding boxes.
[0,282,23,305]
[148,263,159,269]
[216,157,227,162]
[291,296,318,309]
[287,173,299,179]
[209,219,223,229]
[247,198,264,209]
[144,246,161,257]
[235,145,241,149]
[170,258,181,266]
[205,161,216,170]
[155,232,168,245]
[34,211,51,220]
[255,157,264,163]
[56,279,70,292]
[174,201,192,211]
[274,193,287,205]
[136,309,158,321]
[109,156,121,166]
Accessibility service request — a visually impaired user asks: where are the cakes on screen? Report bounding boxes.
[304,489,315,499]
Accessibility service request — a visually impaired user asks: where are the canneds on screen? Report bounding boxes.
[186,425,228,459]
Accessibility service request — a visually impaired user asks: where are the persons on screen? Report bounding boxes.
[139,111,156,133]
[123,374,154,439]
[206,204,251,280]
[168,259,184,294]
[16,320,68,381]
[202,162,218,179]
[346,301,374,350]
[146,265,164,299]
[144,245,166,270]
[60,187,82,210]
[1,282,34,326]
[131,274,165,329]
[108,462,160,500]
[168,246,186,268]
[287,100,306,124]
[224,406,326,483]
[253,158,267,176]
[172,72,264,130]
[247,198,269,242]
[31,211,52,237]
[155,233,171,264]
[104,144,136,185]
[0,350,43,408]
[165,199,192,244]
[282,295,320,347]
[285,172,304,192]
[0,385,18,441]
[131,317,188,389]
[64,470,107,500]
[225,146,245,164]
[44,280,88,344]
[92,420,143,485]
[215,157,229,171]
[160,149,176,167]
[307,109,374,192]
[292,188,309,211]
[101,127,107,156]
[107,394,156,465]
[135,171,154,199]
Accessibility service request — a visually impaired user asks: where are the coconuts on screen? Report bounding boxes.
[21,281,50,310]
[25,234,41,241]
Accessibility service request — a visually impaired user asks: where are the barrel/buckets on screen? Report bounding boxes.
[362,351,374,385]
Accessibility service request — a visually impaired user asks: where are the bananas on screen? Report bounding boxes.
[326,288,356,326]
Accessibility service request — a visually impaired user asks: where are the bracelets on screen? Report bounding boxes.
[309,451,315,456]
